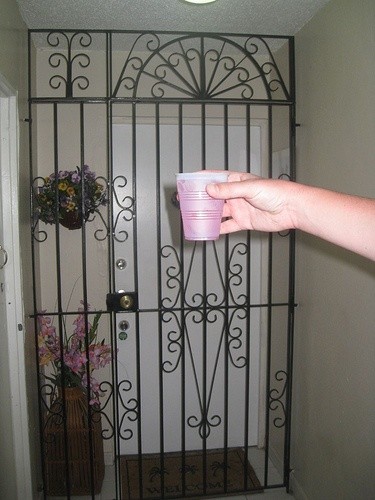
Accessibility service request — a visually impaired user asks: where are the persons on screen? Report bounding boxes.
[174,170,375,265]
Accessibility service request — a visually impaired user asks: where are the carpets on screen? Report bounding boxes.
[117,448,263,500]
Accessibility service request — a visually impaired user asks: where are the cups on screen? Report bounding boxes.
[176,171,230,240]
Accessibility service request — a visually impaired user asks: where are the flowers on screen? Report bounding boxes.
[36,300,109,393]
[36,162,105,230]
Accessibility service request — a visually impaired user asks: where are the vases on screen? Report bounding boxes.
[35,384,107,497]
